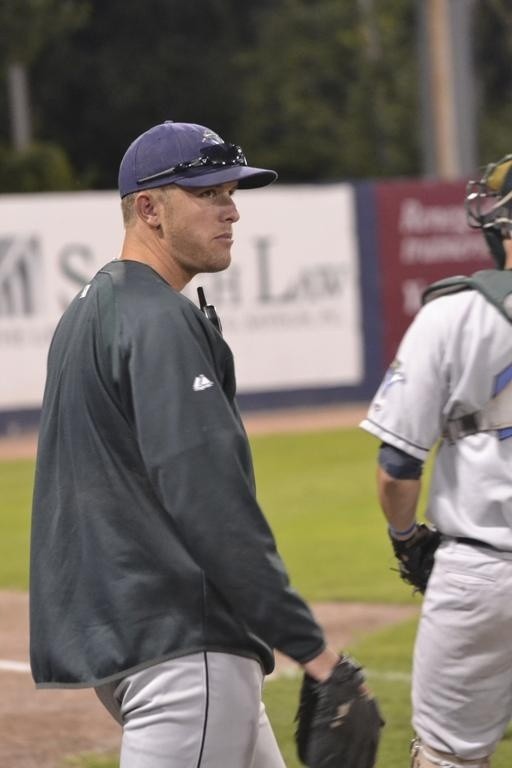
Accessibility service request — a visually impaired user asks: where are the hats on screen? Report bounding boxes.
[118,119,280,200]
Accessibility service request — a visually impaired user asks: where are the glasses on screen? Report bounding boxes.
[137,142,248,185]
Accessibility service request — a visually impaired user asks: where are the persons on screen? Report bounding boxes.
[33,119,390,768]
[353,147,512,768]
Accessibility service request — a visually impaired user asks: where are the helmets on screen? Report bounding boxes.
[464,152,512,267]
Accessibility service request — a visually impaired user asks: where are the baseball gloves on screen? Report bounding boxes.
[293,651,385,768]
[388,522,441,595]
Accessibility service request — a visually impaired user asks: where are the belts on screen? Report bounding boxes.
[454,536,512,553]
